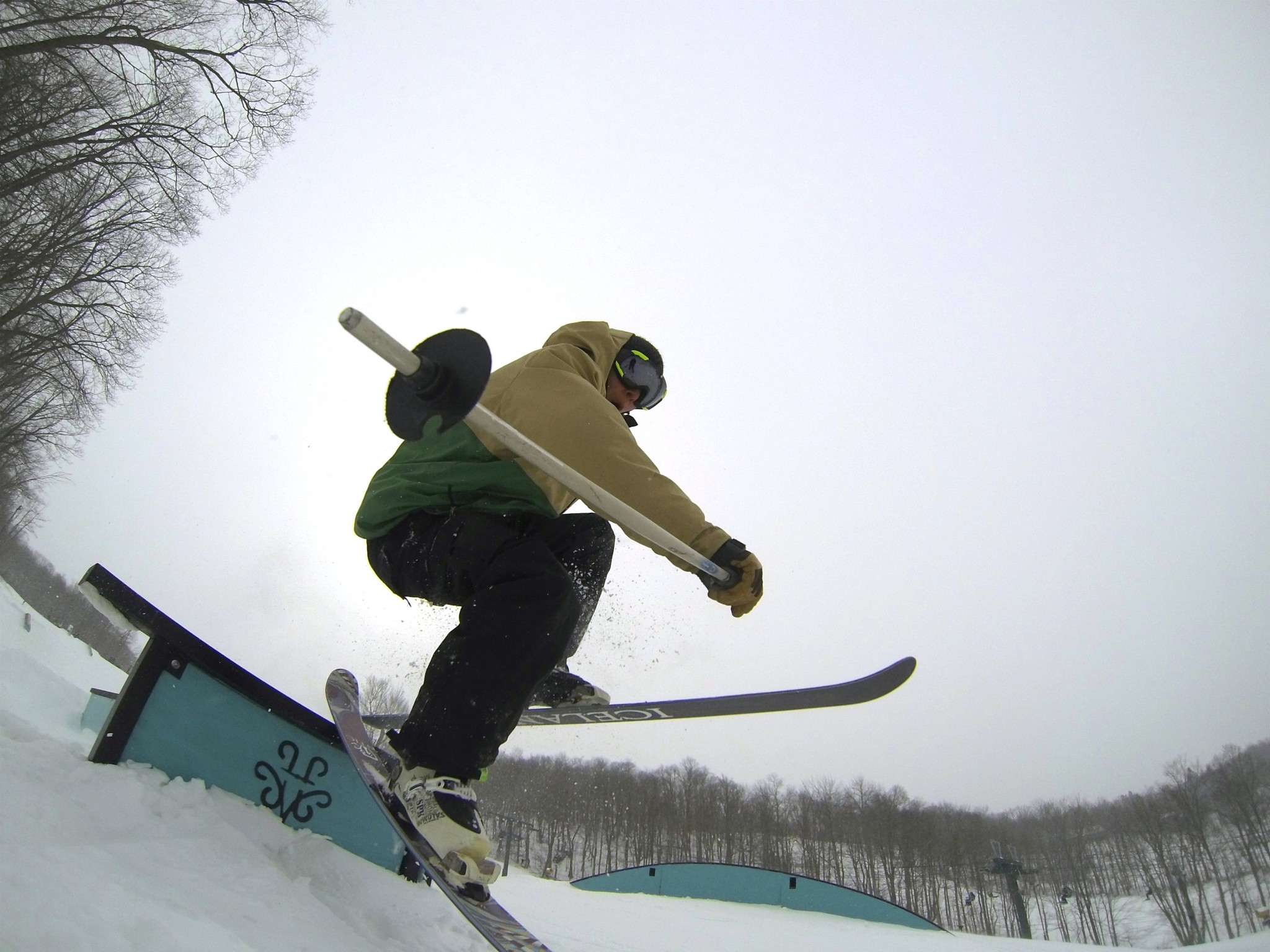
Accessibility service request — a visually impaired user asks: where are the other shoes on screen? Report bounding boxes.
[390,761,492,862]
[527,668,594,705]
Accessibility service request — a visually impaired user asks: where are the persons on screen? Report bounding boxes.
[352,320,764,864]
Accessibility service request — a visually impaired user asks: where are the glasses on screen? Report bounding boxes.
[615,352,667,410]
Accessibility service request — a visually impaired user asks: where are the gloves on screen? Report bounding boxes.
[698,541,763,618]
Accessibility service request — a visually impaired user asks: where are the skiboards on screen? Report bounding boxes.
[325,657,918,952]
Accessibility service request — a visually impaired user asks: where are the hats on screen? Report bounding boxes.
[622,333,665,375]
